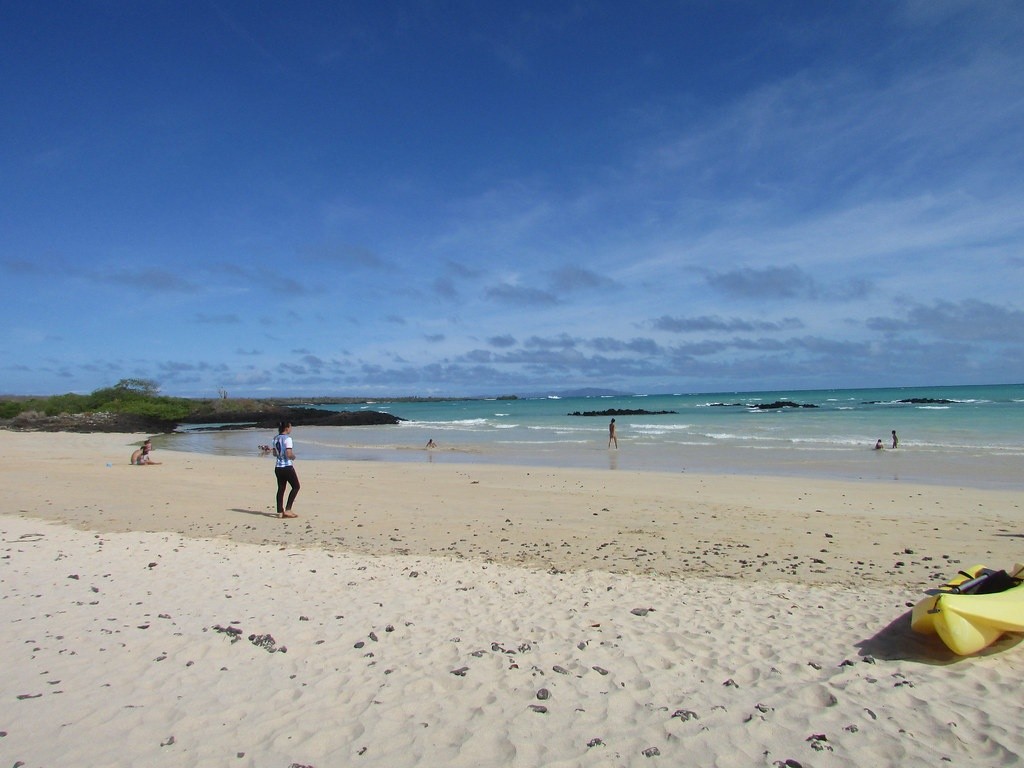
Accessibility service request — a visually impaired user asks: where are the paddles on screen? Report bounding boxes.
[923,567,998,596]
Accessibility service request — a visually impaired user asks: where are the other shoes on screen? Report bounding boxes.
[284,512,297,518]
[279,513,283,517]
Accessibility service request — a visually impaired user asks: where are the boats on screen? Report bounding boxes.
[911,562,1024,655]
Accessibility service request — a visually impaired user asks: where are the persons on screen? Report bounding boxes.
[426,439,437,448]
[131,439,161,465]
[273,422,300,517]
[259,445,273,452]
[892,430,898,448]
[876,439,883,449]
[609,418,618,448]
